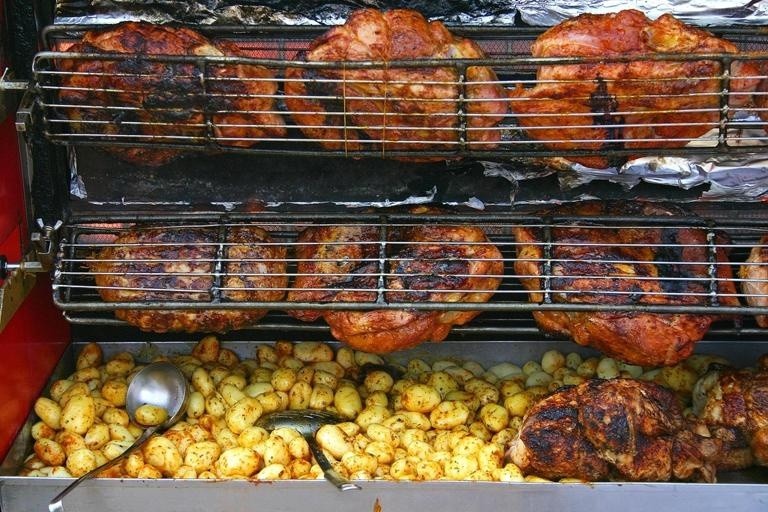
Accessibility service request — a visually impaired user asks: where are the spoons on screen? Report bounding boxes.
[49,360,189,512]
[253,407,368,492]
